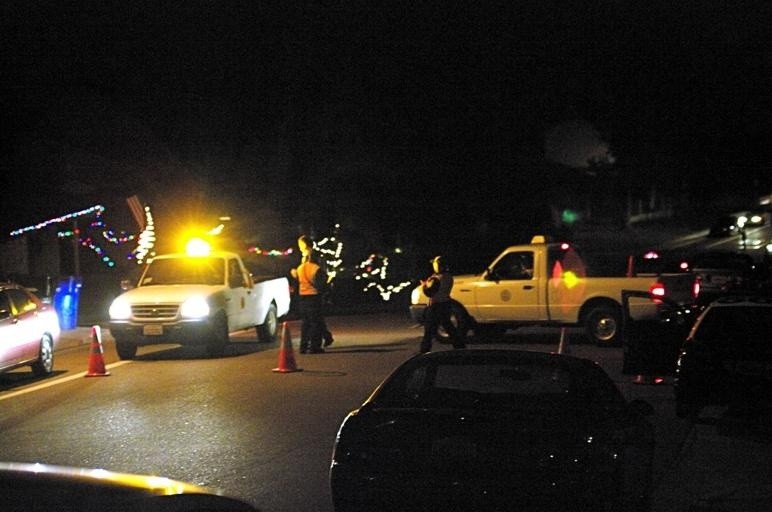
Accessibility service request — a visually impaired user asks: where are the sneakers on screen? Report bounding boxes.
[299,337,334,354]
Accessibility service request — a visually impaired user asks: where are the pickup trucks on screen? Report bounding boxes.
[408,233,703,348]
[107,248,293,362]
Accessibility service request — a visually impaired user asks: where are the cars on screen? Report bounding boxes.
[593,247,772,434]
[327,349,656,511]
[0,457,257,511]
[0,283,59,379]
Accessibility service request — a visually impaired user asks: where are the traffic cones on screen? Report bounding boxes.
[84,325,111,377]
[273,321,304,374]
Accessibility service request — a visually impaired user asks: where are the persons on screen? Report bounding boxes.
[296,246,328,356]
[417,255,467,354]
[518,255,534,279]
[290,234,335,347]
